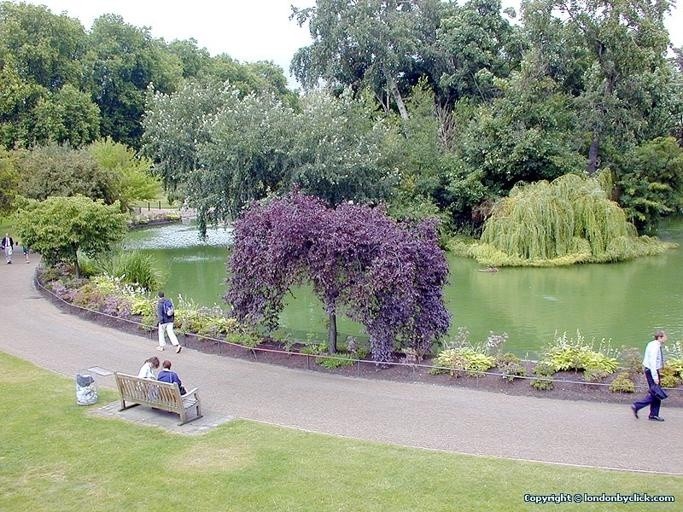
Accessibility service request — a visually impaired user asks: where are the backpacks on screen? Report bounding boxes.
[164,299,174,317]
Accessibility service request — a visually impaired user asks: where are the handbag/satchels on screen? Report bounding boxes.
[648,384,668,401]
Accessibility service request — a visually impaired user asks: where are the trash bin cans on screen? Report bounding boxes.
[76,370,97,406]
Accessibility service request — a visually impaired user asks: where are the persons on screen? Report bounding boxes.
[1,233,14,264]
[631,329,668,421]
[157,360,186,413]
[156,291,182,353]
[137,357,160,410]
[23,246,30,263]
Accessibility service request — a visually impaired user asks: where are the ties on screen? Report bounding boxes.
[660,346,664,370]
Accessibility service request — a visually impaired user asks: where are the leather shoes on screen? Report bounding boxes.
[649,414,664,421]
[632,404,639,418]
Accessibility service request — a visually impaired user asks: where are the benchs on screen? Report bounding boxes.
[114,370,203,426]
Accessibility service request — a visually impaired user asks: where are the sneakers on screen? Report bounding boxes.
[156,344,182,353]
